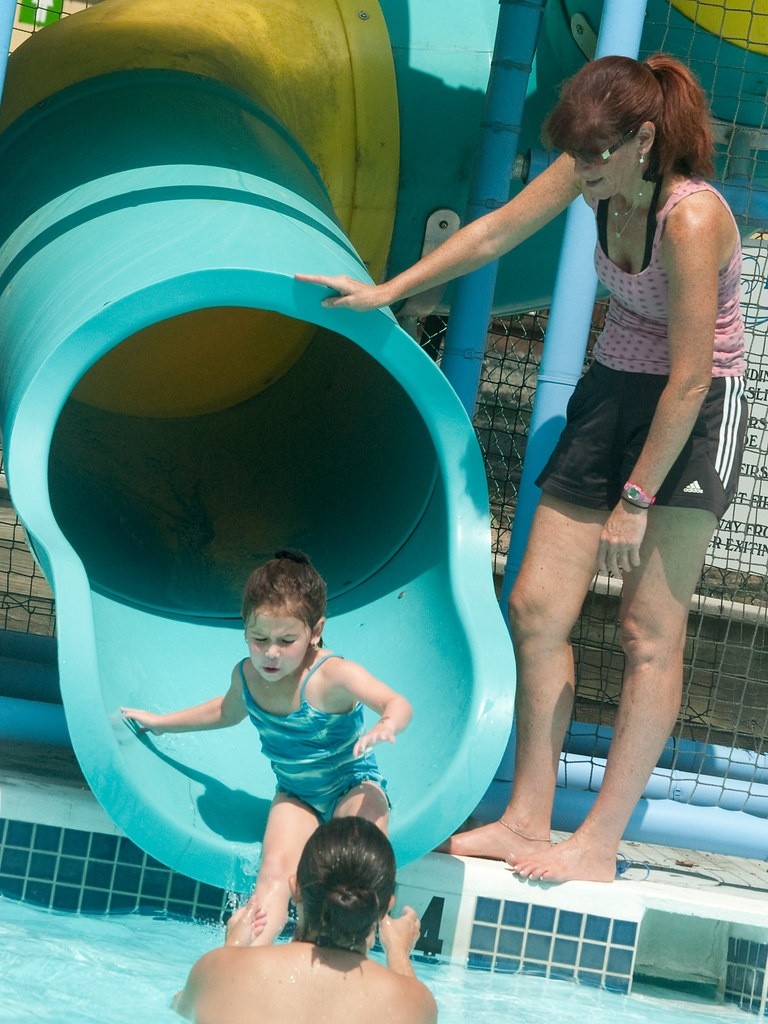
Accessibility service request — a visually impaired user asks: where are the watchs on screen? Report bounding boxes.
[624,482,654,502]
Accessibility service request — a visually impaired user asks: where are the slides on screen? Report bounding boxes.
[1,0,768,904]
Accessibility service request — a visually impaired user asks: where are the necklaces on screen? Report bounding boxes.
[612,182,646,239]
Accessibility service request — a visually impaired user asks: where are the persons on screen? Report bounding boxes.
[294,54,748,882]
[168,814,436,1024]
[120,552,413,950]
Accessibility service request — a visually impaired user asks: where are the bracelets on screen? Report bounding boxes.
[618,495,650,510]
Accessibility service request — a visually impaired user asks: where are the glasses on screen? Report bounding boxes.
[565,127,638,166]
